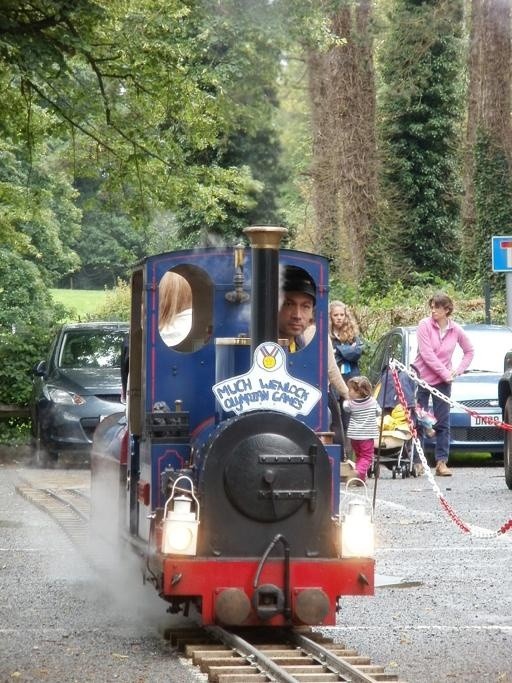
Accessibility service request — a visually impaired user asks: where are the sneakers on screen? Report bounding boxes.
[415,463,424,476]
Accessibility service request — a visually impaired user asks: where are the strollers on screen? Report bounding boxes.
[367,359,423,478]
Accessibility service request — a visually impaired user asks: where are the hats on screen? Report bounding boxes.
[283,265,316,307]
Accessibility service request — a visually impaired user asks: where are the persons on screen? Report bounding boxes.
[308,307,349,401]
[328,300,364,484]
[412,294,475,476]
[277,266,316,353]
[341,374,381,485]
[147,271,191,347]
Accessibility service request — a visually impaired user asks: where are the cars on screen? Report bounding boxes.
[30,321,135,467]
[370,325,512,463]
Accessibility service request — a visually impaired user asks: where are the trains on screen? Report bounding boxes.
[92,225,374,632]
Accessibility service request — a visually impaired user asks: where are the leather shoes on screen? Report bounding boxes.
[436,459,452,476]
